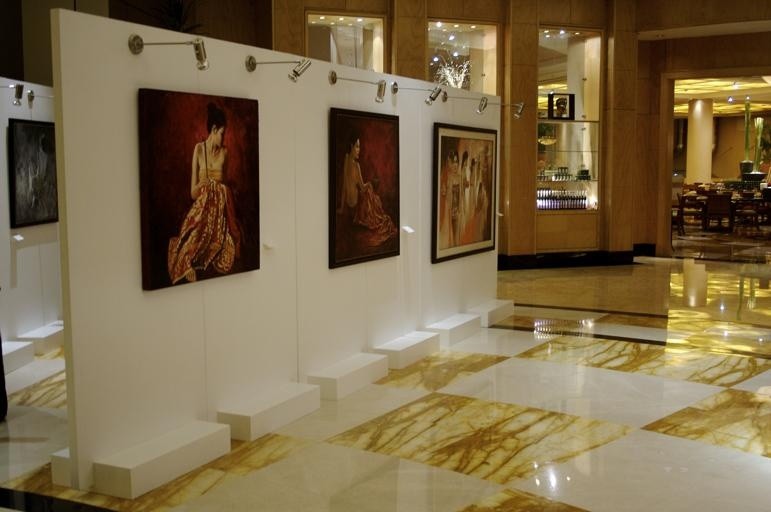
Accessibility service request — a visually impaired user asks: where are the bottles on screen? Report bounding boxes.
[537,165,592,209]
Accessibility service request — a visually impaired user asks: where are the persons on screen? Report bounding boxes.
[335,117,397,247]
[166,102,247,285]
[443,144,491,248]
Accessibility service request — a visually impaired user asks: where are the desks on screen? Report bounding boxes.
[538,169,558,181]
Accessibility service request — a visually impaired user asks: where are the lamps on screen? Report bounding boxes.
[537,135,557,146]
[442,91,488,114]
[328,71,386,103]
[0,83,23,105]
[245,55,312,82]
[487,101,527,120]
[129,33,210,71]
[390,81,441,106]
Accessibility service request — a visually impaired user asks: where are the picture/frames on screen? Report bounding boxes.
[432,121,496,263]
[8,117,58,229]
[329,106,400,269]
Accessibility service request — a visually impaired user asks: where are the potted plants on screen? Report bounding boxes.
[739,100,768,181]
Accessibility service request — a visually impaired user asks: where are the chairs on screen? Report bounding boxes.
[537,166,549,181]
[554,166,572,180]
[574,168,591,180]
[672,175,771,236]
